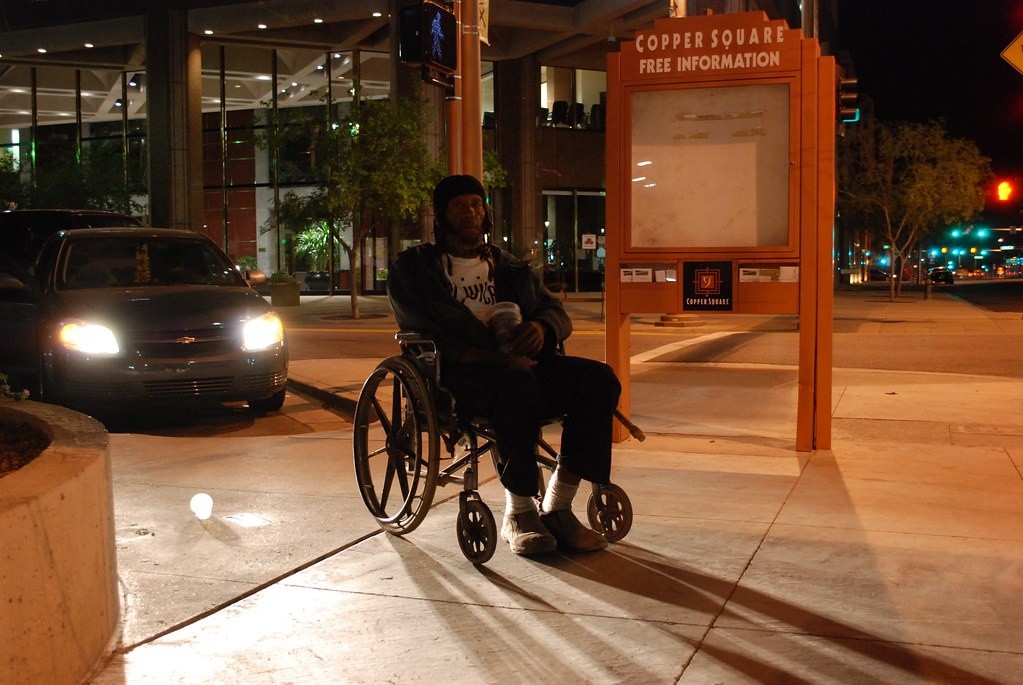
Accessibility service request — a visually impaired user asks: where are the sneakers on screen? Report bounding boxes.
[537,502,608,552]
[500,509,557,554]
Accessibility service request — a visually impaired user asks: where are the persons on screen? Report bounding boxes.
[386,174,622,555]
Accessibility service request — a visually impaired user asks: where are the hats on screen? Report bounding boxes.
[433,174,486,211]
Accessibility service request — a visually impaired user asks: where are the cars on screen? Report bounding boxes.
[930,266,955,284]
[0,226,289,418]
[0,208,146,376]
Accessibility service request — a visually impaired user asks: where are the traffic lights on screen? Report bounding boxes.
[942,247,948,254]
[993,176,1018,205]
[834,64,860,138]
[970,246,978,254]
[400,0,459,77]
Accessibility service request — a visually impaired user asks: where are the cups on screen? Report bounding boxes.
[486,301,521,352]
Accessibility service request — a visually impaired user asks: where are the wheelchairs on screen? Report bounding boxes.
[353,332,646,566]
